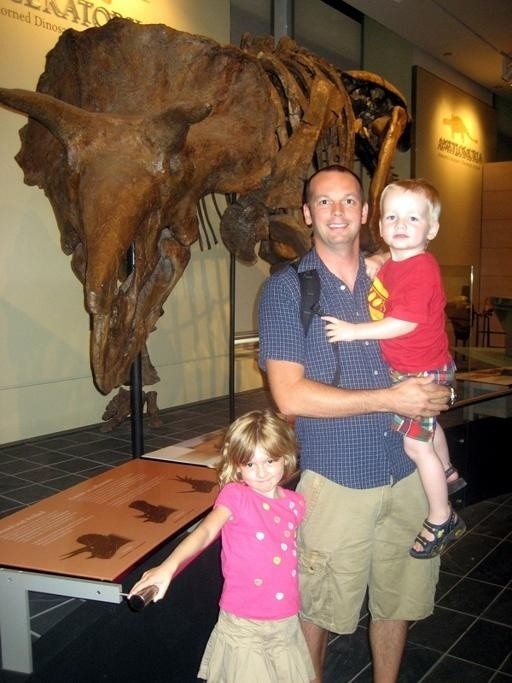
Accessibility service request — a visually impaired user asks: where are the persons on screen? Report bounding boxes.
[251,164,459,683]
[445,285,476,360]
[125,407,318,683]
[317,178,470,560]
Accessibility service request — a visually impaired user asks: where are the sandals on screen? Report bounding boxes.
[443,466,467,496]
[410,504,469,558]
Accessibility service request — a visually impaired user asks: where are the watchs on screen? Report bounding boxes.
[446,384,460,407]
